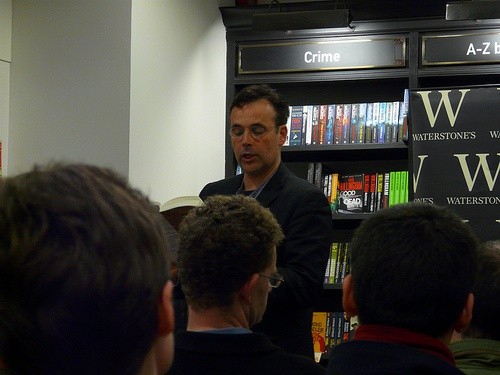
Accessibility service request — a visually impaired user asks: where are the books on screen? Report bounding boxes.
[320,241,354,285]
[277,87,410,147]
[234,161,409,216]
[306,309,362,363]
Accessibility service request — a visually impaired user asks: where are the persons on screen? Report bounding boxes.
[0,160,176,375]
[199,83,330,359]
[325,203,478,375]
[163,194,330,375]
[450,240,500,375]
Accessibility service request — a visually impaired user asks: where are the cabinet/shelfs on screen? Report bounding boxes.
[219,0,500,359]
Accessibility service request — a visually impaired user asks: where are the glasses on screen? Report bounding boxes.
[249,272,285,288]
[229,126,276,138]
